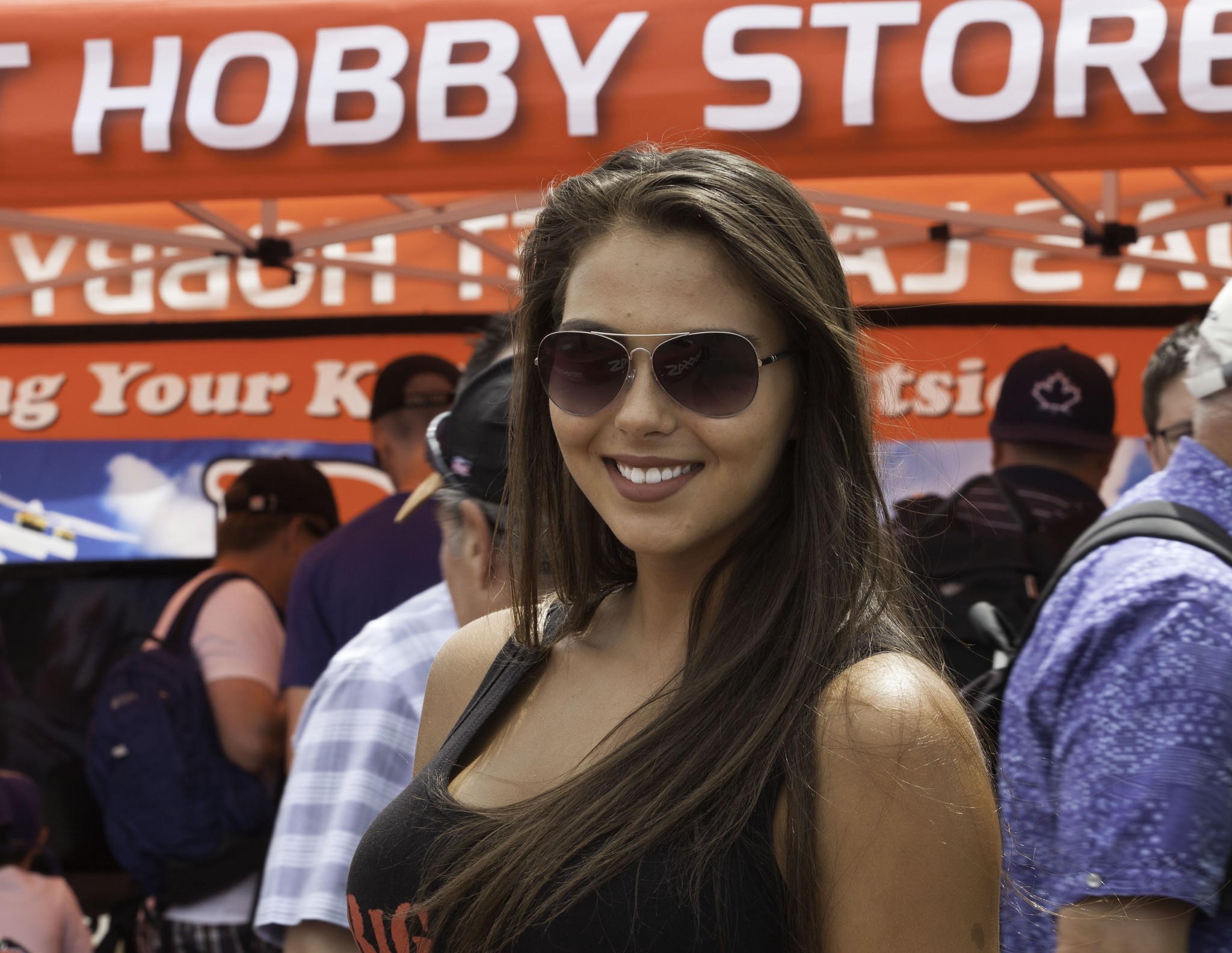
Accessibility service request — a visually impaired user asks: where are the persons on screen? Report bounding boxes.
[344,142,1002,953]
[0,320,552,953]
[883,274,1232,953]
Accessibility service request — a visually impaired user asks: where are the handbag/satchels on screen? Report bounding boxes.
[85,572,285,901]
[906,475,1109,741]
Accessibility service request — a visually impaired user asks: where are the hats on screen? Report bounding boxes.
[396,348,552,547]
[1183,278,1232,399]
[989,344,1116,451]
[225,455,339,535]
[368,353,462,422]
[0,769,42,823]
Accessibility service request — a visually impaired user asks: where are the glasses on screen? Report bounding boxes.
[533,330,798,418]
[1155,419,1192,454]
